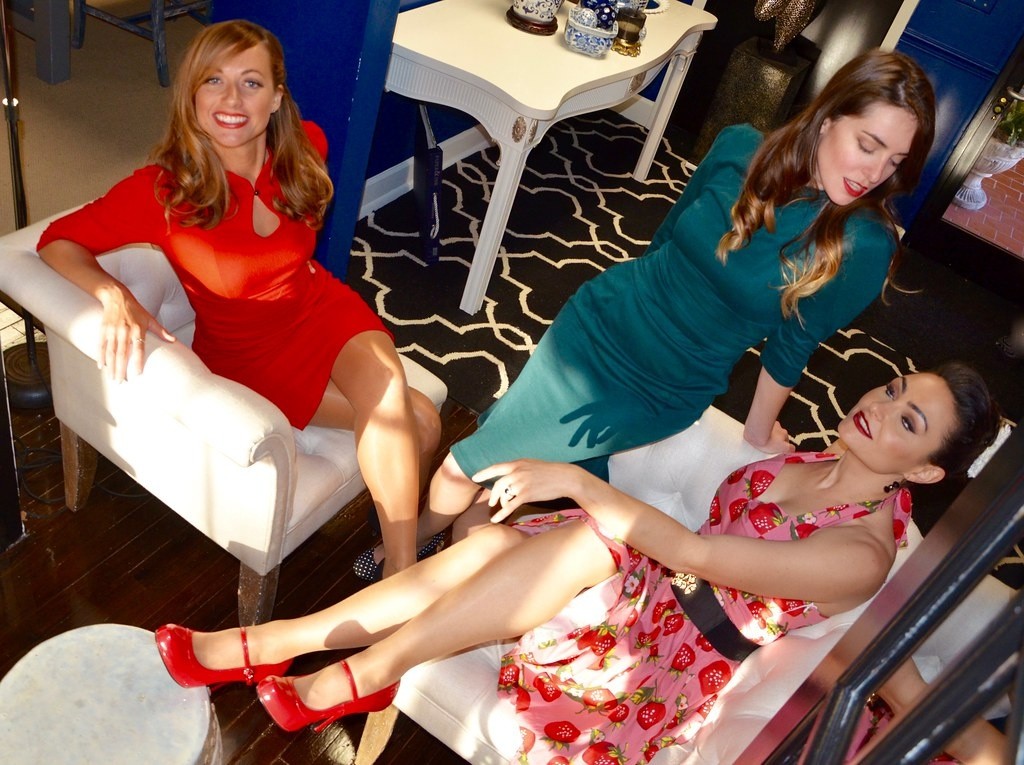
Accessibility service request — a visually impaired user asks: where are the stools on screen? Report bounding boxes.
[1,623,224,765]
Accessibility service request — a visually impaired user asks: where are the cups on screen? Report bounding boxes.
[611,8,646,57]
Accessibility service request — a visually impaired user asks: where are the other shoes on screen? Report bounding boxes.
[353,529,447,582]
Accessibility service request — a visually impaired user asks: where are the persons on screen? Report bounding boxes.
[155,366,1002,764]
[37,17,442,585]
[352,50,936,588]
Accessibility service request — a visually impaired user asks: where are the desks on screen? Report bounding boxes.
[386,0,718,314]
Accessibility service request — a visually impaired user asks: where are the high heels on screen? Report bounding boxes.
[154,622,294,692]
[256,660,401,732]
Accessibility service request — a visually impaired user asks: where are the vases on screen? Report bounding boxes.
[952,126,1024,210]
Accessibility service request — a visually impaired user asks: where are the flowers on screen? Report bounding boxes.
[1000,99,1024,147]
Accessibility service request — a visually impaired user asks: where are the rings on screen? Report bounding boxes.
[504,486,514,496]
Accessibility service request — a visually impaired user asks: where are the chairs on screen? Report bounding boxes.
[0,197,449,628]
[352,403,923,765]
